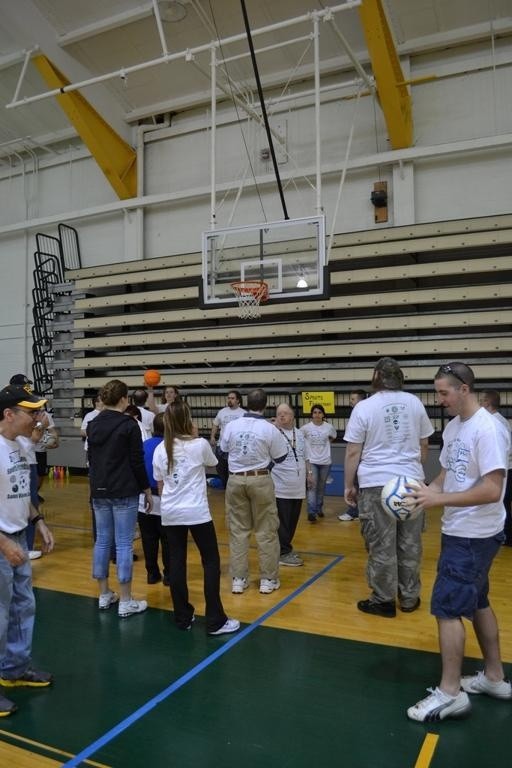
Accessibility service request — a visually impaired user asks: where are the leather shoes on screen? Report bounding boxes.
[308,514,318,522]
[162,576,170,584]
[357,598,399,619]
[113,555,139,563]
[402,598,422,612]
[147,573,159,583]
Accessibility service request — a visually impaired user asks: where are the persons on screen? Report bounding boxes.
[33,404,60,505]
[343,357,435,618]
[80,394,104,451]
[0,383,56,716]
[479,387,511,545]
[123,404,147,443]
[219,388,289,594]
[144,381,183,415]
[9,373,48,559]
[210,390,249,484]
[152,400,241,636]
[86,380,154,618]
[130,388,155,443]
[271,402,314,567]
[141,412,167,585]
[337,389,370,522]
[298,404,338,522]
[399,361,512,724]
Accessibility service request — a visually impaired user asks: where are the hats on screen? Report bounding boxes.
[9,374,35,388]
[1,384,49,409]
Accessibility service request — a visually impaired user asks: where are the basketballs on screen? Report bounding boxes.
[143,368,160,387]
[380,475,427,522]
[43,433,57,448]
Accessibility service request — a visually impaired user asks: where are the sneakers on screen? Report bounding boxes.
[117,601,149,618]
[460,671,511,700]
[2,670,58,688]
[407,690,471,723]
[28,551,42,561]
[100,593,121,608]
[231,577,251,594]
[259,577,282,594]
[279,553,305,568]
[338,513,354,523]
[2,697,20,716]
[207,617,241,637]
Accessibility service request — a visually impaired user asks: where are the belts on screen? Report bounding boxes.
[1,530,28,538]
[228,470,273,477]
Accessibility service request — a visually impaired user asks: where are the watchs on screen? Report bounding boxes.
[33,422,45,432]
[30,514,45,525]
[307,469,313,475]
[30,514,46,522]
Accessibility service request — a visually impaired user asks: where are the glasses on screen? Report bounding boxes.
[13,405,42,420]
[438,361,471,389]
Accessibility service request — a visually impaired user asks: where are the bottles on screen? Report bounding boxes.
[49,465,69,479]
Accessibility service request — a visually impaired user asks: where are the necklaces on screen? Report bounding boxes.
[272,423,305,467]
